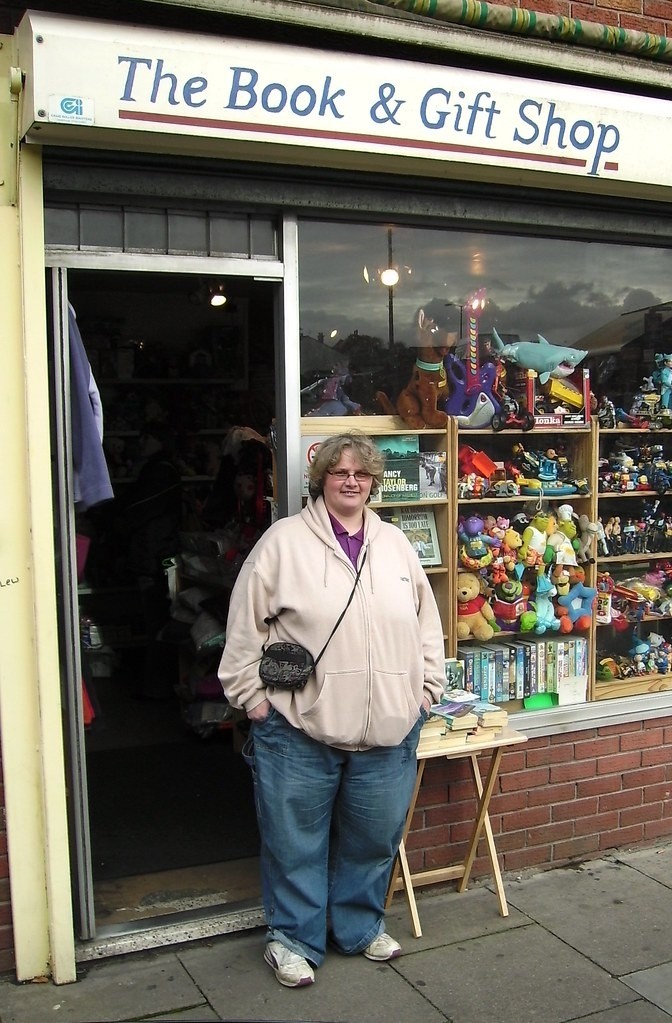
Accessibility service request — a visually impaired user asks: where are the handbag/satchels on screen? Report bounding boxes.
[258,642,314,691]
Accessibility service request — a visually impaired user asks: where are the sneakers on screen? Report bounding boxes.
[361,933,401,961]
[263,938,315,987]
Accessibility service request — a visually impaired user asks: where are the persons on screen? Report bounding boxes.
[421,463,436,486]
[217,433,447,988]
[104,427,193,699]
[616,642,672,680]
[482,337,507,395]
[439,460,446,492]
[596,353,672,557]
[413,535,430,558]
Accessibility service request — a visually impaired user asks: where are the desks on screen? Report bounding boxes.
[384,727,528,939]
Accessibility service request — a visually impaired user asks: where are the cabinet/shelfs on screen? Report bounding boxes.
[300,414,672,716]
[90,343,249,649]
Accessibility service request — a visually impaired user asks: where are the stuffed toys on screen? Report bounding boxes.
[302,308,598,641]
[628,631,665,663]
[225,443,270,561]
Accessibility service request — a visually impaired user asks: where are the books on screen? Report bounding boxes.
[392,505,442,566]
[371,434,420,501]
[419,451,448,500]
[415,633,588,753]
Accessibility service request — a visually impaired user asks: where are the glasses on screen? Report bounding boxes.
[325,468,374,482]
